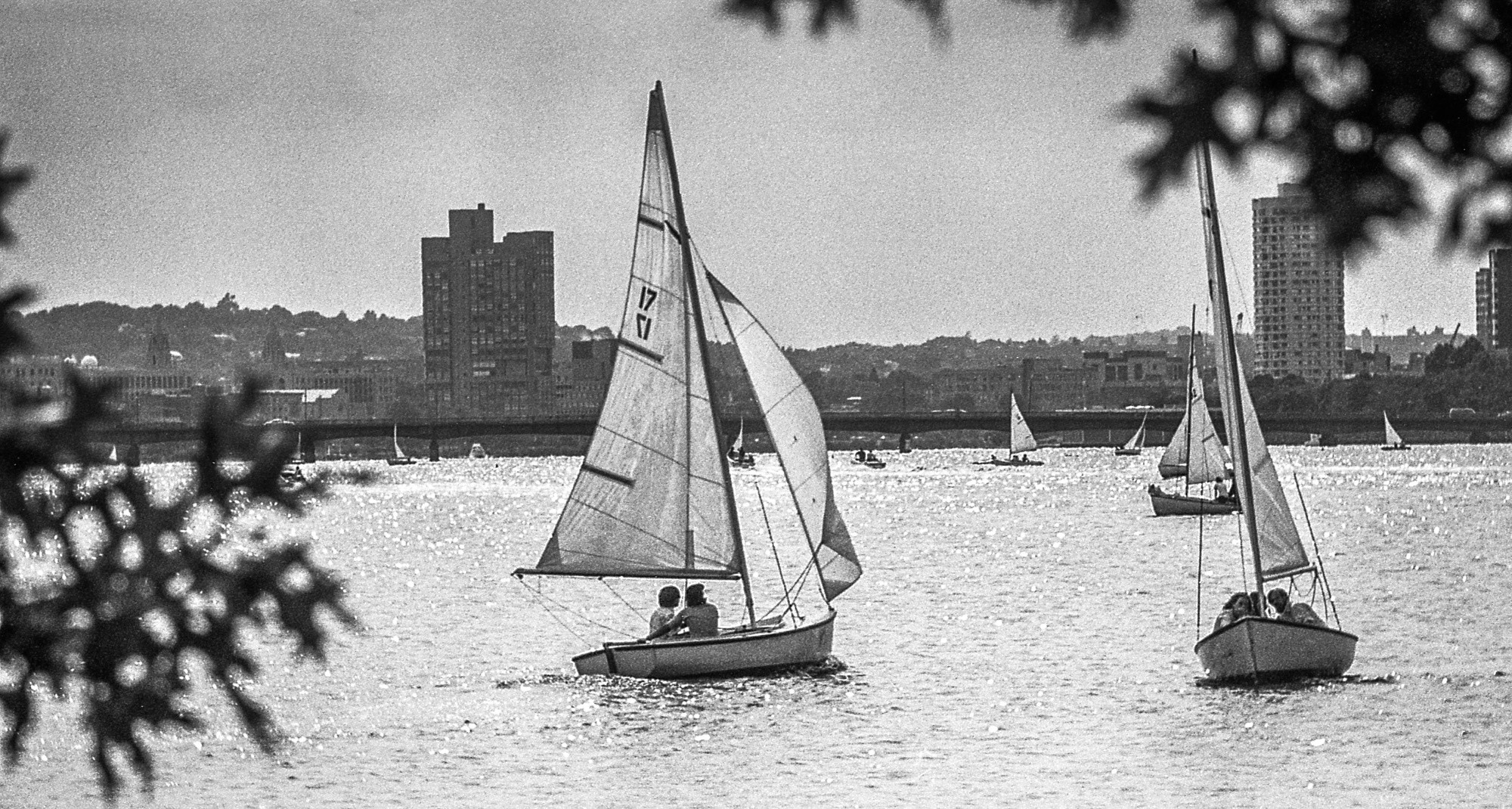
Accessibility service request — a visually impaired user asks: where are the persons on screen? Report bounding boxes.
[637,583,719,644]
[729,445,755,464]
[648,585,690,641]
[991,454,1029,463]
[1213,588,1327,635]
[854,447,878,462]
[1213,471,1243,514]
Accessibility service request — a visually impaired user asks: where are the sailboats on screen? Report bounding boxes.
[385,423,418,465]
[725,416,756,467]
[1193,48,1360,687]
[1114,413,1148,455]
[510,80,864,680]
[1380,410,1412,451]
[993,393,1043,466]
[1148,301,1243,517]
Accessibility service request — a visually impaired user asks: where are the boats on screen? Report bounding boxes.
[278,466,306,486]
[851,458,886,469]
[470,443,486,458]
